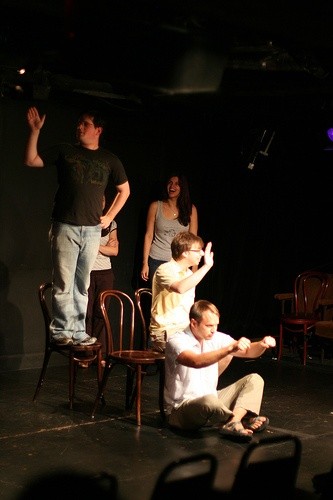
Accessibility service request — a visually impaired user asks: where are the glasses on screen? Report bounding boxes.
[190,249,201,253]
[77,121,95,128]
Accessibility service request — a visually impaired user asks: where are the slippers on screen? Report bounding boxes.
[246,415,270,432]
[220,422,254,441]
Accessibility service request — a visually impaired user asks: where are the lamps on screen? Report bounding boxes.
[258,130,276,156]
[246,127,269,171]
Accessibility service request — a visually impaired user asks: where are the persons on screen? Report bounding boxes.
[86,195,119,363]
[148,232,214,354]
[163,300,277,443]
[141,172,198,351]
[25,106,130,346]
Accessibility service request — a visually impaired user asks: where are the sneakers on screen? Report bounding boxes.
[55,337,74,347]
[79,336,97,346]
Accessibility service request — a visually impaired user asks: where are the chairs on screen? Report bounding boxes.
[228,433,302,500]
[32,280,104,411]
[132,286,167,413]
[273,270,333,366]
[148,451,219,500]
[89,289,168,427]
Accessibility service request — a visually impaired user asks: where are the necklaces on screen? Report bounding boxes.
[167,202,177,218]
